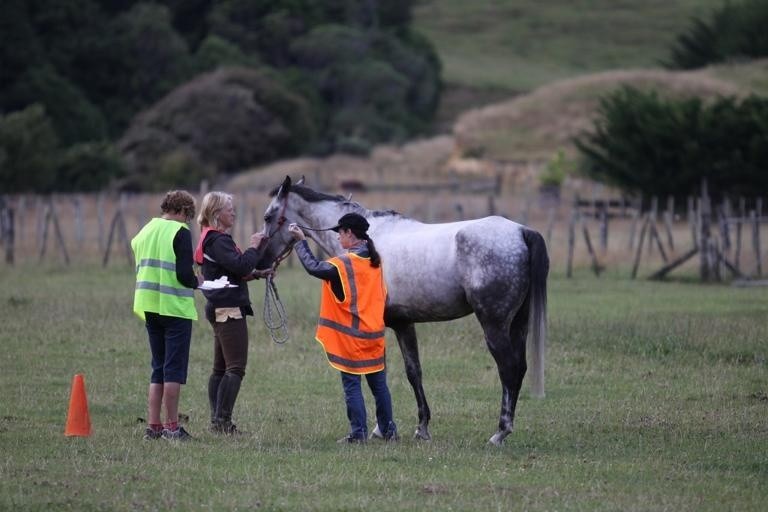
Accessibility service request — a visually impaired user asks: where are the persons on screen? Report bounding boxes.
[130,189,204,445]
[289,212,395,445]
[194,192,277,436]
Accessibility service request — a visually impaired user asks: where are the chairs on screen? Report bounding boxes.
[335,435,396,445]
[142,427,200,444]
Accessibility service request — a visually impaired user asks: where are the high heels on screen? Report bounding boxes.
[65,375,92,438]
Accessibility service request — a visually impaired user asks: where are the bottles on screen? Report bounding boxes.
[331,212,369,233]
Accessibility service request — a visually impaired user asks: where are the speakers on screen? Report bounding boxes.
[207,373,253,437]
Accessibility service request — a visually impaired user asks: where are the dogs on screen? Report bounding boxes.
[254,174,549,448]
